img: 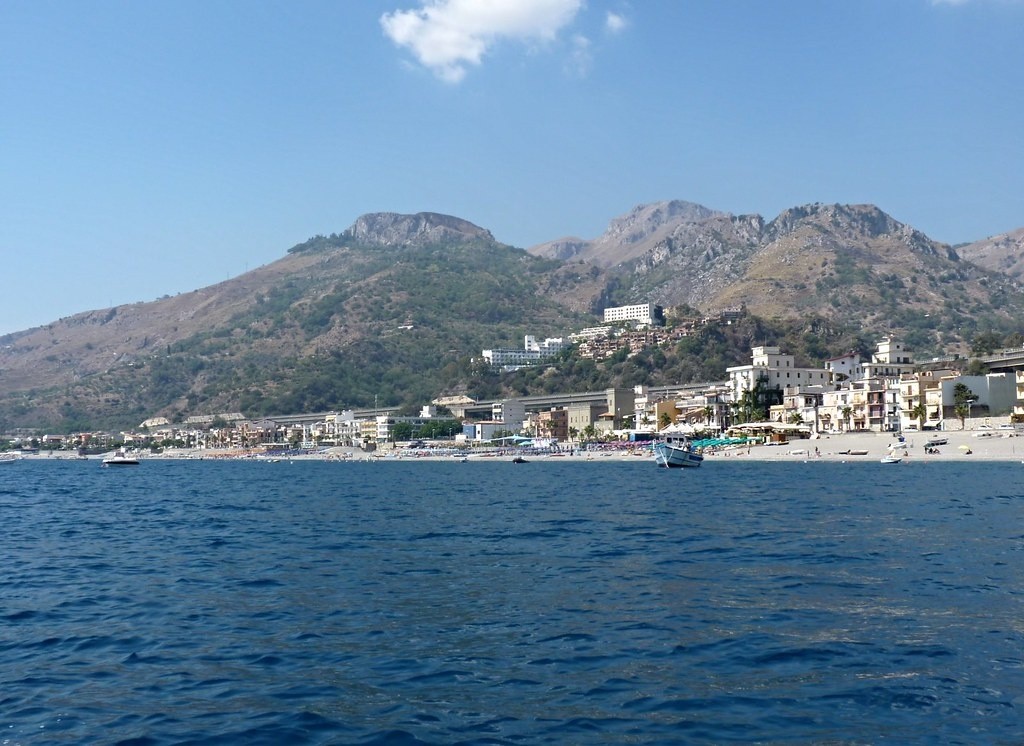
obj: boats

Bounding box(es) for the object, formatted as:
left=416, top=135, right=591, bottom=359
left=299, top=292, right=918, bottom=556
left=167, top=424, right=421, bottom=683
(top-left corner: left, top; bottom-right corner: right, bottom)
left=838, top=449, right=870, bottom=455
left=881, top=456, right=902, bottom=465
left=652, top=439, right=704, bottom=467
left=924, top=436, right=947, bottom=447
left=102, top=450, right=142, bottom=467
left=0, top=451, right=18, bottom=464
left=887, top=441, right=906, bottom=451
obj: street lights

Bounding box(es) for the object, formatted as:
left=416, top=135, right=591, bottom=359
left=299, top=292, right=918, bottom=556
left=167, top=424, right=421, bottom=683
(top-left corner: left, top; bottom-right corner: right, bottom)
left=967, top=399, right=974, bottom=429
left=618, top=407, right=621, bottom=430
left=432, top=428, right=436, bottom=440
left=449, top=428, right=453, bottom=440
left=411, top=430, right=414, bottom=440
